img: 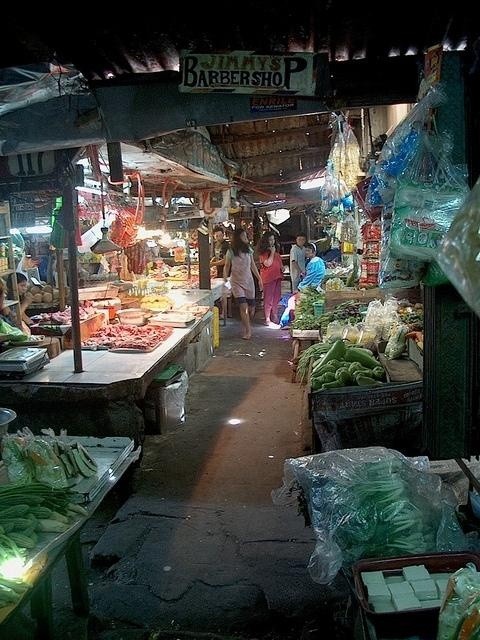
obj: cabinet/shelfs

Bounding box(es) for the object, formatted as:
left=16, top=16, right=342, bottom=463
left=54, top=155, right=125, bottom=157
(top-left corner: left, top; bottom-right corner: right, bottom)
left=0, top=199, right=22, bottom=344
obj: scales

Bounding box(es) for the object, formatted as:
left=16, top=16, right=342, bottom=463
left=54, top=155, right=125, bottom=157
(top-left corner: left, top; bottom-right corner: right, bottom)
left=0, top=346, right=49, bottom=378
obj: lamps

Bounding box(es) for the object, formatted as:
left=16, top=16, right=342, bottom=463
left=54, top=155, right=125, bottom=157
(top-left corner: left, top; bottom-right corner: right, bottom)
left=89, top=174, right=123, bottom=257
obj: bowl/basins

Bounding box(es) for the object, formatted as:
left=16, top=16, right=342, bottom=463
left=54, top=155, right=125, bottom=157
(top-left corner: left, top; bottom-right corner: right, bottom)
left=0, top=406, right=18, bottom=438
left=114, top=308, right=152, bottom=326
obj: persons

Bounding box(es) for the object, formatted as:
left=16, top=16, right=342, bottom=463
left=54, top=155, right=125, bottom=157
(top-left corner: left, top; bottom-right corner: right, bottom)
left=289, top=232, right=308, bottom=294
left=209, top=228, right=233, bottom=319
left=7, top=273, right=34, bottom=326
left=222, top=229, right=264, bottom=340
left=0, top=277, right=27, bottom=343
left=280, top=243, right=326, bottom=330
left=257, top=232, right=285, bottom=325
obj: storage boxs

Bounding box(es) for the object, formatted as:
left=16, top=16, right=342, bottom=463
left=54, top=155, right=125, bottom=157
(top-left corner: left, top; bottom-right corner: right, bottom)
left=30, top=311, right=104, bottom=349
left=351, top=549, right=480, bottom=631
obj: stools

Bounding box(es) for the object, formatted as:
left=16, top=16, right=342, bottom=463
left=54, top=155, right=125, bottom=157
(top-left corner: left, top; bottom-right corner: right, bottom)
left=291, top=328, right=321, bottom=383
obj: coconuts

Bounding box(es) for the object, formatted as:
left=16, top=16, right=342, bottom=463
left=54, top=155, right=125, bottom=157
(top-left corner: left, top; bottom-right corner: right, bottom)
left=30, top=284, right=59, bottom=303
